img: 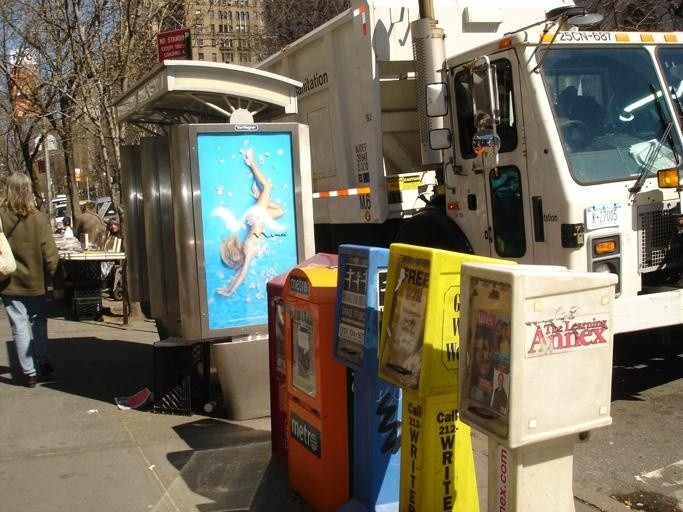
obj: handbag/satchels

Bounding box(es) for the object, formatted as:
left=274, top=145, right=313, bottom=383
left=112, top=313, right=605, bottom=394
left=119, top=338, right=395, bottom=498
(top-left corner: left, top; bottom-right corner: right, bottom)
left=0, top=232, right=17, bottom=282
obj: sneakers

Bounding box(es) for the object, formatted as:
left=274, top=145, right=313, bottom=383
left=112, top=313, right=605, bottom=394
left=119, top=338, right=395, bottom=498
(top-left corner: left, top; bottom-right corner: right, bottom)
left=25, top=373, right=38, bottom=388
left=39, top=364, right=54, bottom=376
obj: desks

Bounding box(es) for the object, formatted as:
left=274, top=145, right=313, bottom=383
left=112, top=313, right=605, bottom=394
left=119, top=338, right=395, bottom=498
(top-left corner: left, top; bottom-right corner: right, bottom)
left=46, top=234, right=129, bottom=326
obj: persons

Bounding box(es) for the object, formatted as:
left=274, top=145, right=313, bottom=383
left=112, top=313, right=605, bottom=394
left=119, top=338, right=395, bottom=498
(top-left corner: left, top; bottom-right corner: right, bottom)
left=106, top=219, right=121, bottom=238
left=73, top=203, right=105, bottom=247
left=63, top=217, right=73, bottom=240
left=0, top=171, right=60, bottom=388
left=216, top=147, right=284, bottom=297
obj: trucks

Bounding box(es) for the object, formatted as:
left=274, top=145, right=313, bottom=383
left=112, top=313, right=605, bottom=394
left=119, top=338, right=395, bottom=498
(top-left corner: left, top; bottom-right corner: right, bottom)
left=253, top=0, right=683, bottom=335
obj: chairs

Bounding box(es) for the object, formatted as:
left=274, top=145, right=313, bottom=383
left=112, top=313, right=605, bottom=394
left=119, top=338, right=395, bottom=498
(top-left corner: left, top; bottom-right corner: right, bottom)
left=74, top=231, right=123, bottom=301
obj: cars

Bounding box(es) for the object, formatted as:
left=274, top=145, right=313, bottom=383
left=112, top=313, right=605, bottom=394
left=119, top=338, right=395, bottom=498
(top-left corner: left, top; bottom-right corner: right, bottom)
left=51, top=195, right=120, bottom=228
